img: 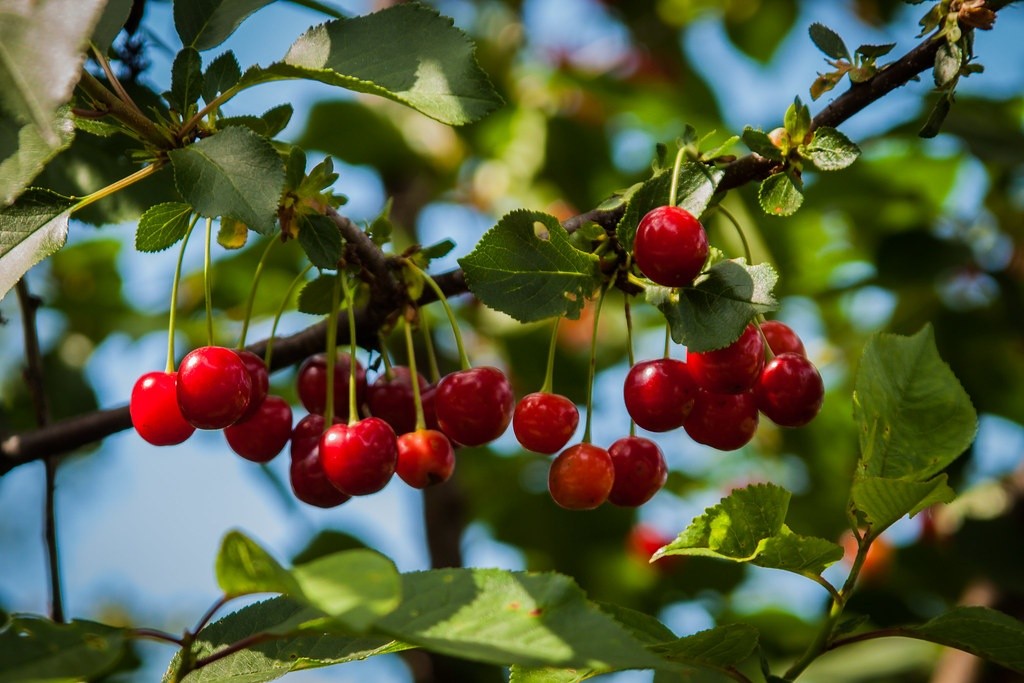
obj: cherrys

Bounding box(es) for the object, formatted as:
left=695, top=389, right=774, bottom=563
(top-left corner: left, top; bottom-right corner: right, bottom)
left=129, top=146, right=825, bottom=511
